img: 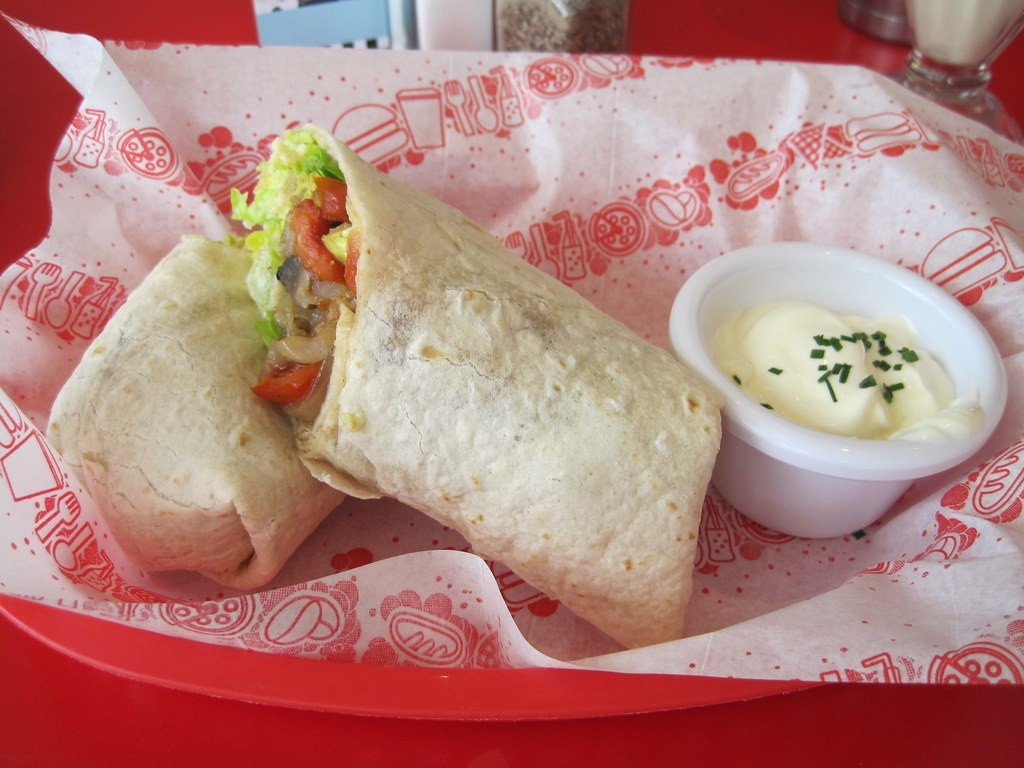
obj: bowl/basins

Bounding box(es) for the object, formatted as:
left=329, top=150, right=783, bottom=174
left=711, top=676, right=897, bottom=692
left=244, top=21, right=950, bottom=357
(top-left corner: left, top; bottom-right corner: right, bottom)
left=670, top=234, right=1007, bottom=538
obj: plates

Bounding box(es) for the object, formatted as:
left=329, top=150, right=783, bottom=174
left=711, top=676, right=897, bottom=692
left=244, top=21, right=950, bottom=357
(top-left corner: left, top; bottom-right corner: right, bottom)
left=0, top=584, right=829, bottom=720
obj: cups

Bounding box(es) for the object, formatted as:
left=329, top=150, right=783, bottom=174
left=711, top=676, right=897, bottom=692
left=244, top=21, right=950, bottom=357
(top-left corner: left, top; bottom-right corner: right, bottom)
left=882, top=1, right=1024, bottom=146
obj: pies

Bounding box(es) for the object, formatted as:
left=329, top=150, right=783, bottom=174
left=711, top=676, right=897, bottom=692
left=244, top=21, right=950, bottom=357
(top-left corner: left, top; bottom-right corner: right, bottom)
left=221, top=122, right=725, bottom=649
left=48, top=232, right=352, bottom=588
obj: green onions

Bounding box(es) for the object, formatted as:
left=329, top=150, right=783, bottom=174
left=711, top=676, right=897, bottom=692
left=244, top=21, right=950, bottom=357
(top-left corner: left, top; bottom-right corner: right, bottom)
left=733, top=331, right=922, bottom=413
